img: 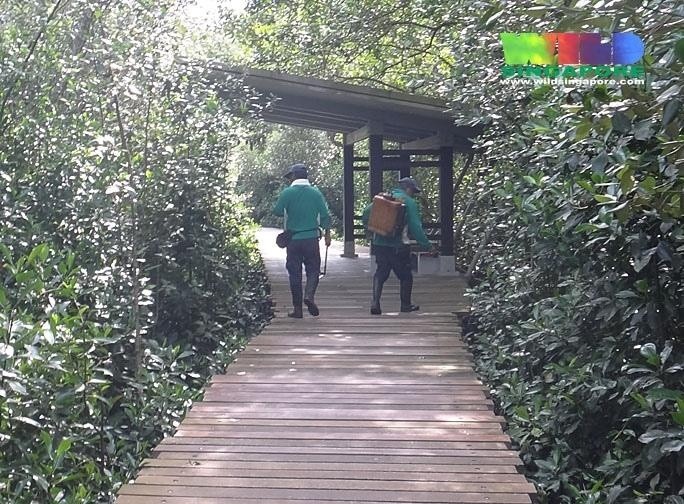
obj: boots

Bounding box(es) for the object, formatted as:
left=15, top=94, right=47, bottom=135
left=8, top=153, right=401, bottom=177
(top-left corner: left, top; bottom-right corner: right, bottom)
left=288, top=304, right=303, bottom=318
left=400, top=280, right=420, bottom=312
left=370, top=280, right=382, bottom=315
left=304, top=286, right=319, bottom=316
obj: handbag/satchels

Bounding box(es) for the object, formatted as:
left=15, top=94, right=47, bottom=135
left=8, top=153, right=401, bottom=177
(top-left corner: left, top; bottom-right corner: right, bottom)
left=276, top=230, right=292, bottom=248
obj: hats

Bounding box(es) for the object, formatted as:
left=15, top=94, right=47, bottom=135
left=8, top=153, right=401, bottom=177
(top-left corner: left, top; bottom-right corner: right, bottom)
left=284, top=164, right=307, bottom=179
left=398, top=177, right=421, bottom=193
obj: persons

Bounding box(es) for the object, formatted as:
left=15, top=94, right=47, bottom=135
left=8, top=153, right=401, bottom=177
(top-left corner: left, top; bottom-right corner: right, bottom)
left=270, top=162, right=331, bottom=318
left=360, top=175, right=441, bottom=315
